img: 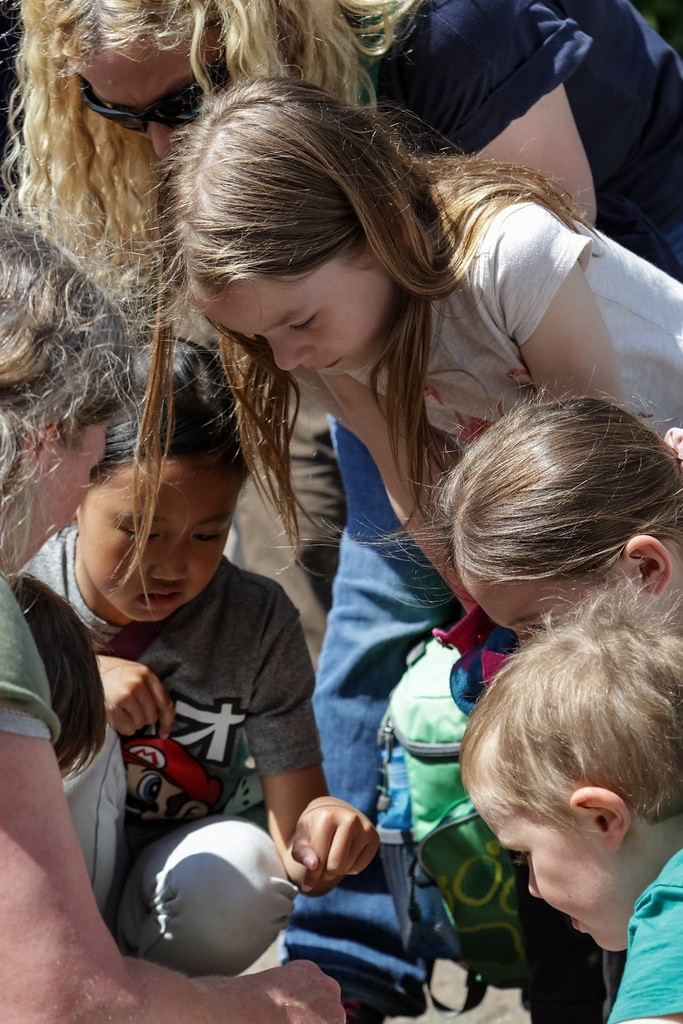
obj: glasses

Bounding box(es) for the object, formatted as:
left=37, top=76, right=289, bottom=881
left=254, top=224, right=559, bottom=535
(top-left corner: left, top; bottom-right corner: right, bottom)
left=73, top=68, right=223, bottom=136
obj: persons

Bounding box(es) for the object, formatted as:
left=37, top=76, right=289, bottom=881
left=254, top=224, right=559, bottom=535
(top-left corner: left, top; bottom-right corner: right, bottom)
left=0, top=0, right=683, bottom=1024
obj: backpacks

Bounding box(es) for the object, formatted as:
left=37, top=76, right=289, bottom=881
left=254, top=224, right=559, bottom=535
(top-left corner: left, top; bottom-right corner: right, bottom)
left=375, top=635, right=543, bottom=991
